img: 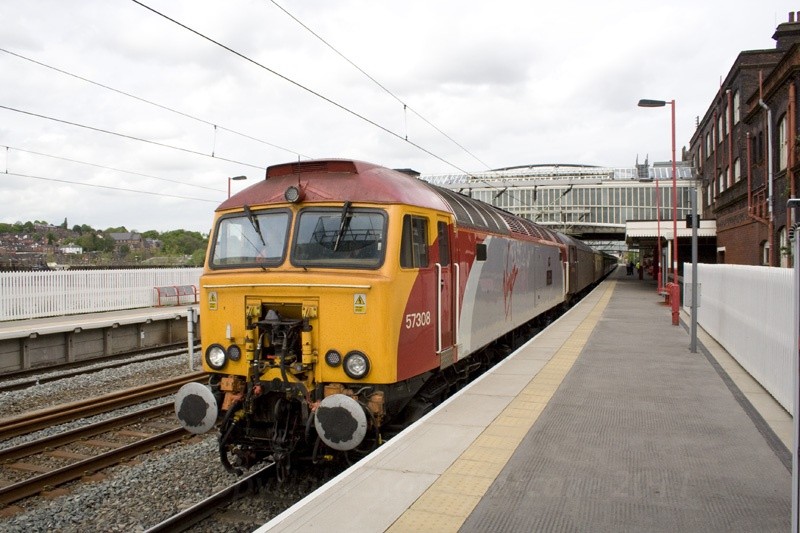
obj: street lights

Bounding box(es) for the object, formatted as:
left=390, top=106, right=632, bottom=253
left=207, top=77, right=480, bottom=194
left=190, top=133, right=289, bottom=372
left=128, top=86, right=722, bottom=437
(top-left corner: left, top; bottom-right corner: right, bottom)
left=228, top=176, right=247, bottom=200
left=638, top=98, right=681, bottom=325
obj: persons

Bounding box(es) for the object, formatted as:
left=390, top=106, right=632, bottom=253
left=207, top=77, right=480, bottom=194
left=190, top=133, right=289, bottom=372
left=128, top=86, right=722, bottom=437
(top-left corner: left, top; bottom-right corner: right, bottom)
left=256, top=228, right=282, bottom=261
left=628, top=260, right=636, bottom=276
left=637, top=265, right=645, bottom=280
left=635, top=259, right=640, bottom=273
left=626, top=262, right=630, bottom=275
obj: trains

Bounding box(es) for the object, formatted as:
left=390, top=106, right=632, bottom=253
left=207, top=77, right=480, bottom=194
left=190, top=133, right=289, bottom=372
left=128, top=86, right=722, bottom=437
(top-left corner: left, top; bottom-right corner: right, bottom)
left=174, top=157, right=620, bottom=484
